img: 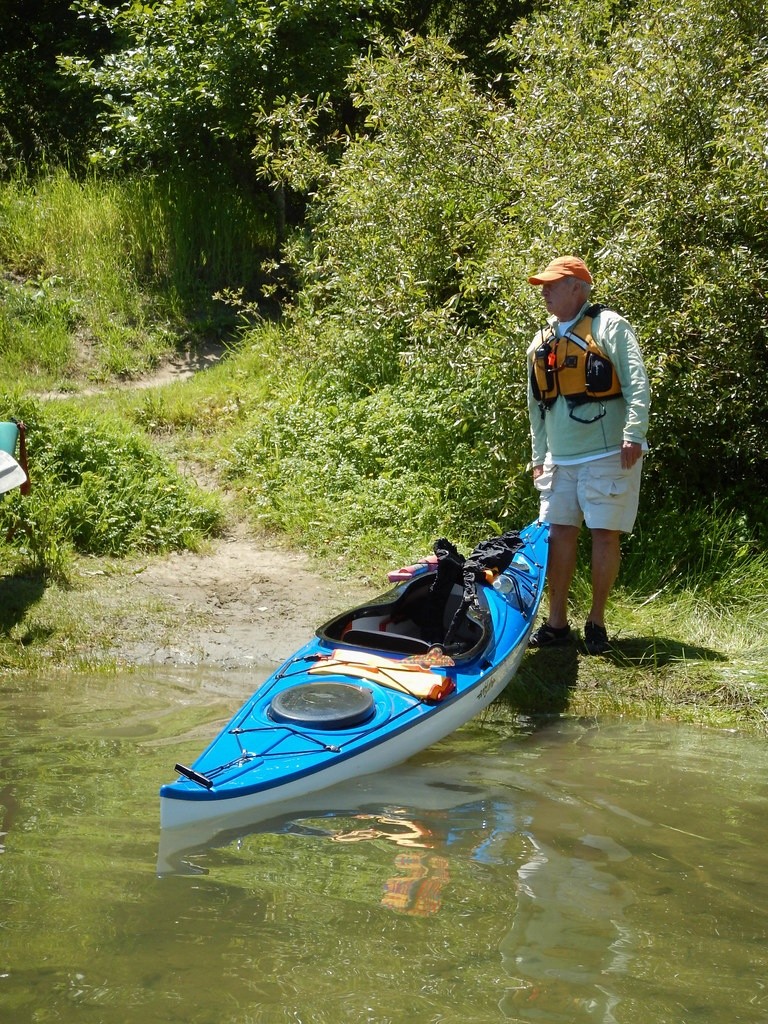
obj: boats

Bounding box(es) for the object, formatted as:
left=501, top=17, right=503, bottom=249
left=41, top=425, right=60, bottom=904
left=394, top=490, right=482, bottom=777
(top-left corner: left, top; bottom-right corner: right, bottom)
left=156, top=516, right=551, bottom=824
left=0, top=421, right=28, bottom=498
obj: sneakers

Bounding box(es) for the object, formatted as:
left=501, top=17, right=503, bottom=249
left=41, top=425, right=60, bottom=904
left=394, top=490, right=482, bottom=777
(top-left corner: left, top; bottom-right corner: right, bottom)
left=584, top=622, right=610, bottom=657
left=527, top=617, right=572, bottom=648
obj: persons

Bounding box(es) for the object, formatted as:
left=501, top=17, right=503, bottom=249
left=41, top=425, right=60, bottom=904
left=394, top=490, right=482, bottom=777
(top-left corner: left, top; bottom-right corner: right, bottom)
left=526, top=256, right=650, bottom=656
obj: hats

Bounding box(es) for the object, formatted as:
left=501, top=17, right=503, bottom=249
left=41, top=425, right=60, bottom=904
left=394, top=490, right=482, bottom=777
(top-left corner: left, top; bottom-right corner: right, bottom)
left=529, top=256, right=592, bottom=285
